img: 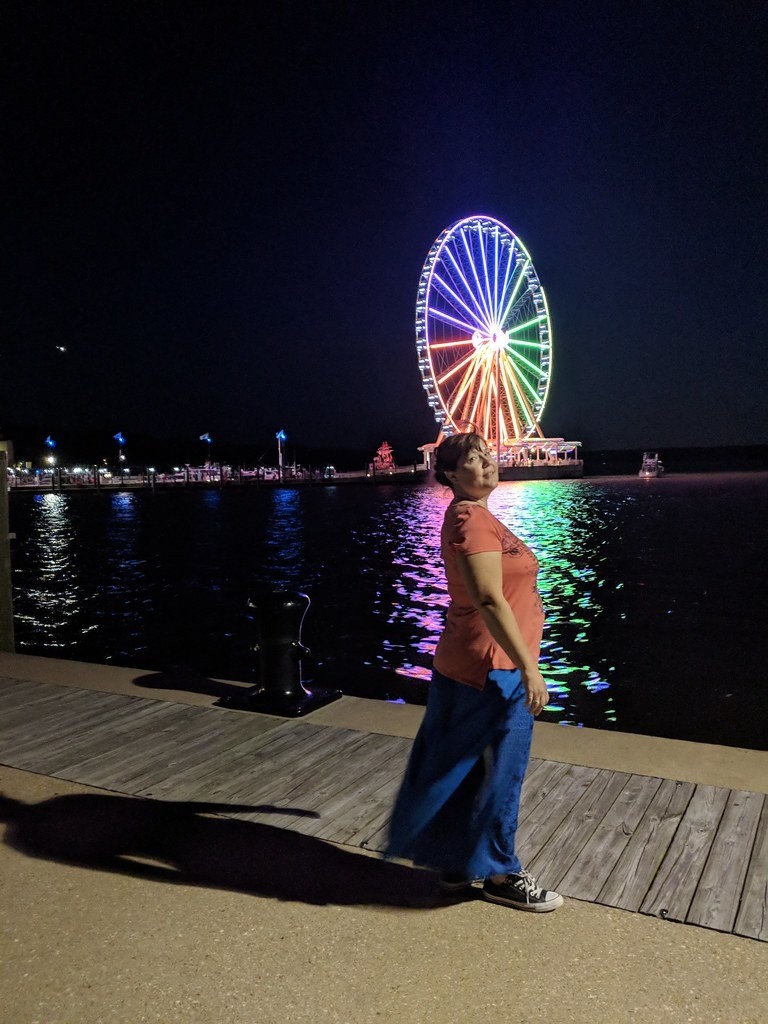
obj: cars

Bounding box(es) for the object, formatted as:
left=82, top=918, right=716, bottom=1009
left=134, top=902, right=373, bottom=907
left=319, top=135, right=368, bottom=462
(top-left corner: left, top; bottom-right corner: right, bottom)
left=321, top=465, right=336, bottom=475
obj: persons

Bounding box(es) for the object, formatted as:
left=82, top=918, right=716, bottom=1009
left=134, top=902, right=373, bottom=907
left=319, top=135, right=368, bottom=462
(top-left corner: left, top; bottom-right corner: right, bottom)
left=387, top=431, right=564, bottom=912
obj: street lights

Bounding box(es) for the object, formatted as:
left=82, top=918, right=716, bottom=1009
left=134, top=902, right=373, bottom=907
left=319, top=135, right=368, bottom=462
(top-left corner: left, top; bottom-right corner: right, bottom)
left=113, top=432, right=125, bottom=486
left=276, top=429, right=286, bottom=483
left=199, top=432, right=211, bottom=481
left=46, top=435, right=55, bottom=490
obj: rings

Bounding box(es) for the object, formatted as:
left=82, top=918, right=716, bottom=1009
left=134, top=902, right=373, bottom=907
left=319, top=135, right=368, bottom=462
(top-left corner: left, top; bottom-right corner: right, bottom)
left=533, top=700, right=540, bottom=704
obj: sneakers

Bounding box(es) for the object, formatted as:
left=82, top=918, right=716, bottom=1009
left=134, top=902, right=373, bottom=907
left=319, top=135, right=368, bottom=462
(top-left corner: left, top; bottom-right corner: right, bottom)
left=441, top=878, right=484, bottom=889
left=482, top=867, right=563, bottom=912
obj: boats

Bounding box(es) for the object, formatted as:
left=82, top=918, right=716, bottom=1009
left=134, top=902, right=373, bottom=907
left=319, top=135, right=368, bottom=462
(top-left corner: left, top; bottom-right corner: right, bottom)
left=639, top=452, right=664, bottom=478
